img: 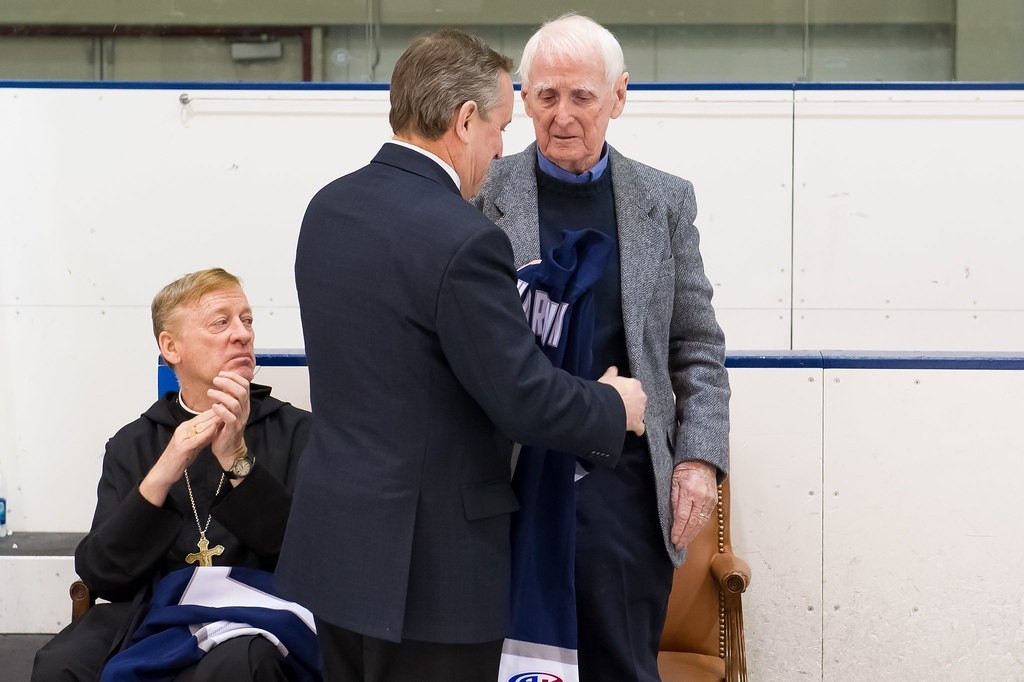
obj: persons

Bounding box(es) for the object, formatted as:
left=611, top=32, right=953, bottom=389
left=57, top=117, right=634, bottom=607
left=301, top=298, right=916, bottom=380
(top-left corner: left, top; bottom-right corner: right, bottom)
left=32, top=269, right=315, bottom=682
left=462, top=11, right=732, bottom=682
left=293, top=31, right=648, bottom=682
left=183, top=467, right=225, bottom=568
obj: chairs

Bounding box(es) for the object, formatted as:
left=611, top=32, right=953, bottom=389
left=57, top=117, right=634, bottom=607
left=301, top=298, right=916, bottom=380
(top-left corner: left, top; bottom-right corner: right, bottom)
left=658, top=477, right=752, bottom=682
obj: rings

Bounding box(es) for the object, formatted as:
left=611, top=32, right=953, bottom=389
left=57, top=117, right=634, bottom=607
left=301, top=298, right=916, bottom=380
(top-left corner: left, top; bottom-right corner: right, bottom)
left=700, top=512, right=709, bottom=519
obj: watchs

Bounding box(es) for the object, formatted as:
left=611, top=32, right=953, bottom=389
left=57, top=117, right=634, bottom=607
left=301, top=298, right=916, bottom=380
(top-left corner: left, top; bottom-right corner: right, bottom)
left=224, top=450, right=257, bottom=479
